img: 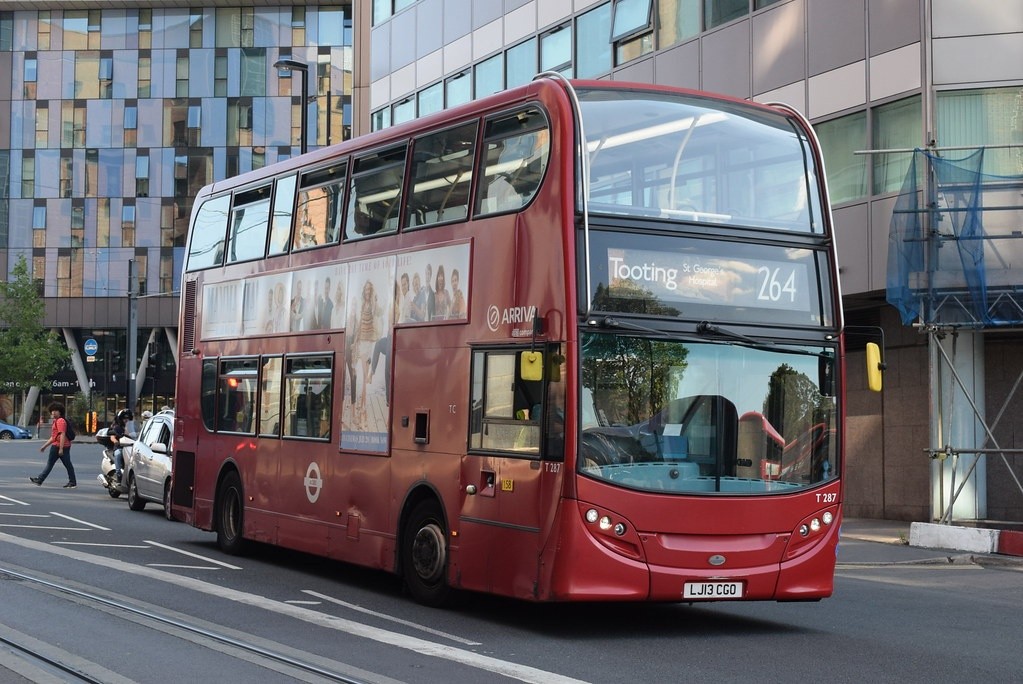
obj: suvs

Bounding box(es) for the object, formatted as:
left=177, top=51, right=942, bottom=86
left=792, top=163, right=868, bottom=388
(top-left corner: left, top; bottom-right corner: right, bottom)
left=126, top=409, right=178, bottom=521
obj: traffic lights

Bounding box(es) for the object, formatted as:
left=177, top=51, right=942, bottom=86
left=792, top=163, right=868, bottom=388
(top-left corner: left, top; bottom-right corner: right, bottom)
left=108, top=349, right=120, bottom=382
left=227, top=377, right=239, bottom=392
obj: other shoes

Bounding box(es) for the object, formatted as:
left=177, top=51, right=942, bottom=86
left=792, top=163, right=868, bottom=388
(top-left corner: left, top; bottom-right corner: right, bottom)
left=117, top=477, right=122, bottom=486
left=63, top=483, right=77, bottom=488
left=30, top=477, right=42, bottom=486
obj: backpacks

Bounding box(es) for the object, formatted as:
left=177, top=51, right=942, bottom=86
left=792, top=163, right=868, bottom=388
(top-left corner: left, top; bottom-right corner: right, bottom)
left=60, top=417, right=76, bottom=442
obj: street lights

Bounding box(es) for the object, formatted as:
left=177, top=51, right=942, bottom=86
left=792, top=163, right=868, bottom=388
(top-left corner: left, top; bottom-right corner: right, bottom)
left=273, top=59, right=309, bottom=154
left=126, top=258, right=140, bottom=410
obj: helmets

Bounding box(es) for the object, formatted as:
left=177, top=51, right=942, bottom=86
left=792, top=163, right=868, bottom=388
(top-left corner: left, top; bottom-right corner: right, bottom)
left=118, top=409, right=133, bottom=426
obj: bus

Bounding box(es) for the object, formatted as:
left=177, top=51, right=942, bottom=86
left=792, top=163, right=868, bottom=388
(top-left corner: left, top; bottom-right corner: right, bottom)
left=168, top=69, right=887, bottom=608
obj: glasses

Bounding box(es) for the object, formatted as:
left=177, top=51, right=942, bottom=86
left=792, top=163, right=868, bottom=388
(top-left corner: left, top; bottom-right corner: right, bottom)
left=122, top=417, right=129, bottom=420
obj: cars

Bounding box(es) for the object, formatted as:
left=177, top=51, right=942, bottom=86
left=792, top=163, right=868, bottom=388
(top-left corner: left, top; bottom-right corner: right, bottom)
left=0, top=420, right=33, bottom=439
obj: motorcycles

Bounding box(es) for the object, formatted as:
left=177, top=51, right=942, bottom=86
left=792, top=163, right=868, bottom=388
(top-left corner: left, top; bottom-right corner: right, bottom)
left=96, top=419, right=142, bottom=499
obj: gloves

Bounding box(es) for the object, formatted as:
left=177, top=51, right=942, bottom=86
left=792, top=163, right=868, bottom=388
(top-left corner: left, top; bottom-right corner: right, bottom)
left=115, top=441, right=121, bottom=449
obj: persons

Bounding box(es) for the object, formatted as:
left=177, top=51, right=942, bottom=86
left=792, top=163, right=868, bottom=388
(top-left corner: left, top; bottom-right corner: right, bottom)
left=261, top=264, right=465, bottom=417
left=533, top=381, right=563, bottom=442
left=315, top=383, right=330, bottom=438
left=29, top=402, right=78, bottom=489
left=107, top=408, right=153, bottom=486
